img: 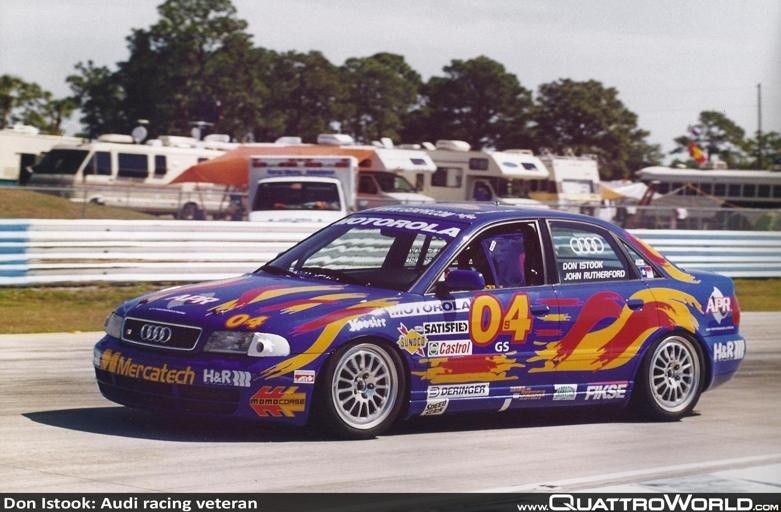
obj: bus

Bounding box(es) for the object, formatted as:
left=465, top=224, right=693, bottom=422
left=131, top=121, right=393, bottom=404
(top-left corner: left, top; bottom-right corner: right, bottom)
left=633, top=164, right=781, bottom=215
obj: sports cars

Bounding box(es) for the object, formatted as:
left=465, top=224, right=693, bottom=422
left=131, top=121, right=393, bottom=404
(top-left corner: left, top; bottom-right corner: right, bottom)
left=88, top=200, right=746, bottom=439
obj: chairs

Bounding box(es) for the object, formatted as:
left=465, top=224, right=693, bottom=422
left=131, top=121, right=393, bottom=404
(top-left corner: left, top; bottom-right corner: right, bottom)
left=448, top=268, right=484, bottom=294
